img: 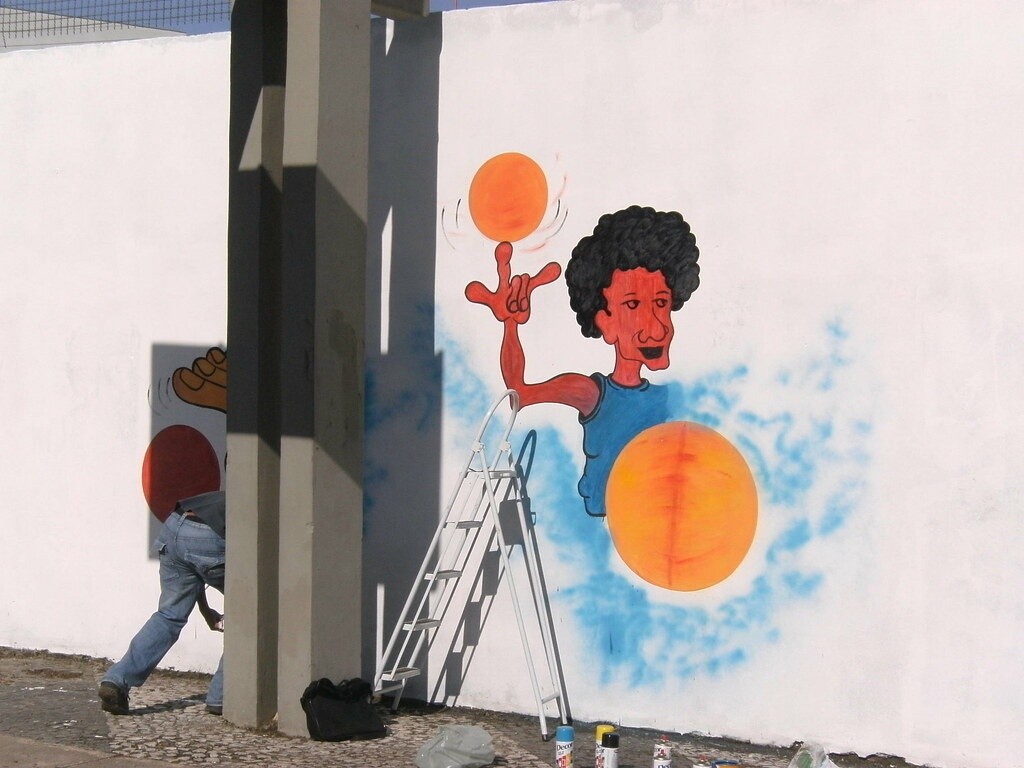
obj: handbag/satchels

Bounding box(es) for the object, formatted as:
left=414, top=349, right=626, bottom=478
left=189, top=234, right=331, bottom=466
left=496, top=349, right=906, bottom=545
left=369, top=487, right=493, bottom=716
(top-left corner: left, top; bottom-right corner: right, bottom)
left=298, top=674, right=386, bottom=741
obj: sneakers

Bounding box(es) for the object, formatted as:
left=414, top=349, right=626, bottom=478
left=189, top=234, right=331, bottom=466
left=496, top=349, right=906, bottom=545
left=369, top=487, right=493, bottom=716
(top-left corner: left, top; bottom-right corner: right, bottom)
left=98, top=683, right=129, bottom=715
left=205, top=704, right=225, bottom=715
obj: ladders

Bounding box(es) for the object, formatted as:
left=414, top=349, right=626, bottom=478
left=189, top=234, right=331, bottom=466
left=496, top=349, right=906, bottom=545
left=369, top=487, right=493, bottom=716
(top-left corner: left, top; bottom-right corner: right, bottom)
left=368, top=389, right=573, bottom=744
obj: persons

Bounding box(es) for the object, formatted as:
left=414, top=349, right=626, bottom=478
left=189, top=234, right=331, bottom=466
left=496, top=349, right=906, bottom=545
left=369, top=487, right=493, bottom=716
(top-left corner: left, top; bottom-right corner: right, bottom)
left=98, top=490, right=228, bottom=714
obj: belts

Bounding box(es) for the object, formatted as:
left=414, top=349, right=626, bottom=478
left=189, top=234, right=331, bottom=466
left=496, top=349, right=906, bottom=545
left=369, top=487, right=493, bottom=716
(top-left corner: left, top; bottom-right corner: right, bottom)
left=174, top=509, right=206, bottom=523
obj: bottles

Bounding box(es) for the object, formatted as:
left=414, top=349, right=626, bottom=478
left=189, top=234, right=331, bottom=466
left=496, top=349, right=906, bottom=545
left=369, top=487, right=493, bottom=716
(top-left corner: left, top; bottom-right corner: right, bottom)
left=594, top=724, right=614, bottom=768
left=692, top=755, right=741, bottom=767
left=654, top=754, right=671, bottom=768
left=654, top=735, right=672, bottom=759
left=555, top=726, right=574, bottom=768
left=601, top=732, right=619, bottom=768
